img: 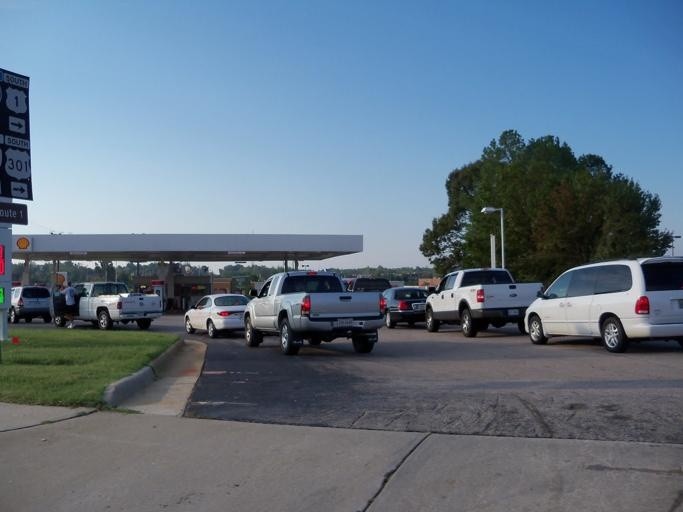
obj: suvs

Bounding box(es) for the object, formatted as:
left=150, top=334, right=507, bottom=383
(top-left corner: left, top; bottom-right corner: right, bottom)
left=7, top=286, right=51, bottom=324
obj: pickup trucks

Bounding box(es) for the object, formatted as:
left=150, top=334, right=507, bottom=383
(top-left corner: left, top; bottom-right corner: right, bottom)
left=423, top=268, right=544, bottom=337
left=49, top=281, right=163, bottom=330
left=241, top=270, right=385, bottom=356
left=340, top=277, right=392, bottom=293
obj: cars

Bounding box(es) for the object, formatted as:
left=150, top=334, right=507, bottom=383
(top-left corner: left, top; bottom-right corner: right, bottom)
left=381, top=287, right=430, bottom=329
left=183, top=294, right=250, bottom=339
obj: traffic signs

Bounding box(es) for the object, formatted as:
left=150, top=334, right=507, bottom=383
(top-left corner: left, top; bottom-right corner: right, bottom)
left=0, top=202, right=29, bottom=226
left=0, top=65, right=32, bottom=200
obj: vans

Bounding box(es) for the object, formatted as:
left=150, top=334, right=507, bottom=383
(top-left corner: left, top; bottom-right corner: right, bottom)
left=523, top=256, right=682, bottom=353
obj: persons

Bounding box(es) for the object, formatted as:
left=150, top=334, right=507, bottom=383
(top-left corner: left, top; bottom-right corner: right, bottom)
left=59, top=280, right=77, bottom=329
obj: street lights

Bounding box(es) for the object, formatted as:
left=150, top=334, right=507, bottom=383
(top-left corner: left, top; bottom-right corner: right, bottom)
left=670, top=235, right=680, bottom=254
left=481, top=207, right=504, bottom=270
